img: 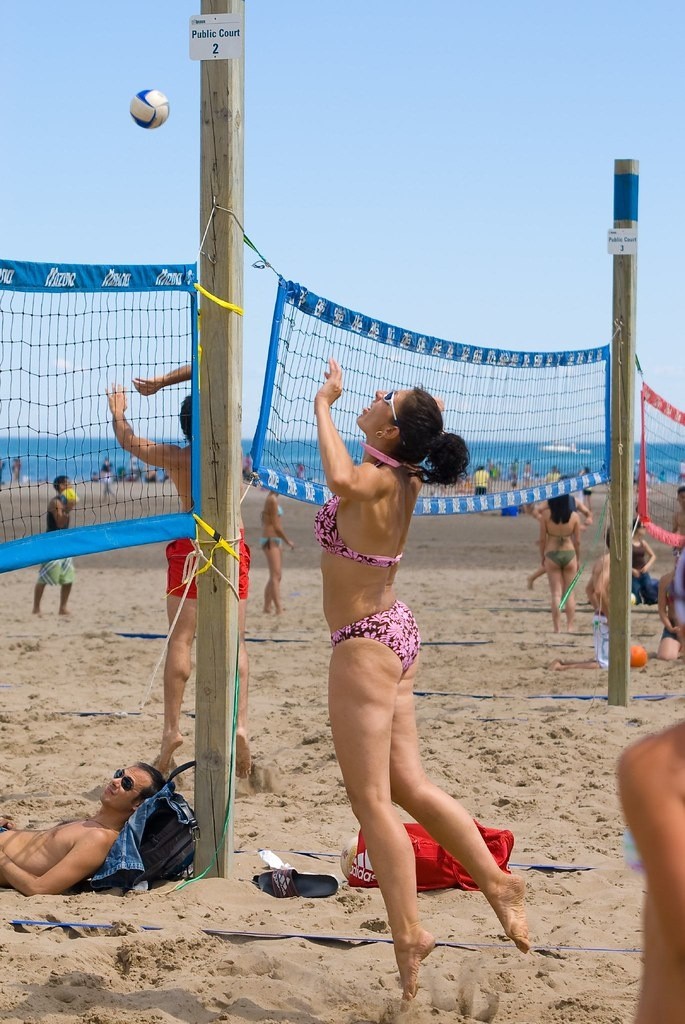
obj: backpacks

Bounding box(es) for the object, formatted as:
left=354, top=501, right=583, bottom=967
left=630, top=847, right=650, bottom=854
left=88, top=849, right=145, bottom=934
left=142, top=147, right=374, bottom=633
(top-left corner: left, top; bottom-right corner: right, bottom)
left=70, top=807, right=196, bottom=894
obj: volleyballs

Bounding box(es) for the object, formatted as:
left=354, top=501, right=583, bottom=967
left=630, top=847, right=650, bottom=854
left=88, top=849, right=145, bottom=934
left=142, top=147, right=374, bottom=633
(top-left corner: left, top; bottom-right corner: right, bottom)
left=130, top=89, right=172, bottom=131
left=61, top=487, right=79, bottom=506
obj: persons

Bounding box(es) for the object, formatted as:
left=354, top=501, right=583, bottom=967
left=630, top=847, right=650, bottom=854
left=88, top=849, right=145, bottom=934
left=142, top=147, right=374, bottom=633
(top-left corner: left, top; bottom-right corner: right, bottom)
left=33, top=476, right=80, bottom=614
left=671, top=487, right=685, bottom=565
left=257, top=489, right=296, bottom=616
left=540, top=495, right=582, bottom=633
left=617, top=719, right=685, bottom=1024
left=0, top=760, right=167, bottom=898
left=313, top=359, right=530, bottom=1001
left=587, top=517, right=685, bottom=659
left=106, top=362, right=252, bottom=778
left=0, top=452, right=685, bottom=517
left=526, top=475, right=593, bottom=591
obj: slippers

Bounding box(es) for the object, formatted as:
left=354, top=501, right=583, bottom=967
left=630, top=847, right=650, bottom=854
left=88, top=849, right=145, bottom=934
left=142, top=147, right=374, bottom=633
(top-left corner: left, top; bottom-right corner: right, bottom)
left=258, top=870, right=339, bottom=898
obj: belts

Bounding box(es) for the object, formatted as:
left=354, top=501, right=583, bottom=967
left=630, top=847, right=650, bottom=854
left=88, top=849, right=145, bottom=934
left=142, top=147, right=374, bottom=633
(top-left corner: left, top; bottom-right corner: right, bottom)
left=166, top=760, right=201, bottom=843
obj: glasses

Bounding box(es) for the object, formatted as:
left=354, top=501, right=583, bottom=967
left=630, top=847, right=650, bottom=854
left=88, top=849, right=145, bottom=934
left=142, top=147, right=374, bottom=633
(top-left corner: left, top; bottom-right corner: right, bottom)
left=383, top=390, right=405, bottom=446
left=114, top=769, right=149, bottom=798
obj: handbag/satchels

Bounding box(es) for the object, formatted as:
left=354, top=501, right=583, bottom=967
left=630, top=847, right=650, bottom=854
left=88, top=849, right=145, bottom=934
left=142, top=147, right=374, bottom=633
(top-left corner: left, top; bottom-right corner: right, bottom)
left=348, top=818, right=515, bottom=891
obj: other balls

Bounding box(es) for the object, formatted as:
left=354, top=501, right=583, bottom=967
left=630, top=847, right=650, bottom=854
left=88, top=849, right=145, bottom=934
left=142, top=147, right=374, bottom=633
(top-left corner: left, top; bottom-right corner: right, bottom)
left=630, top=642, right=648, bottom=666
